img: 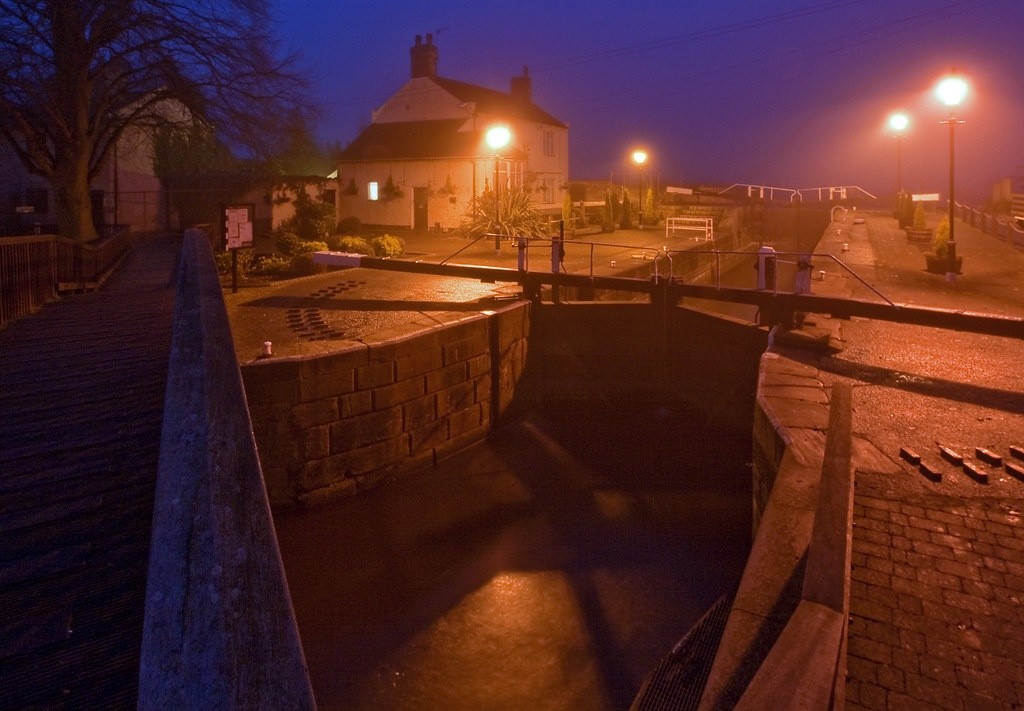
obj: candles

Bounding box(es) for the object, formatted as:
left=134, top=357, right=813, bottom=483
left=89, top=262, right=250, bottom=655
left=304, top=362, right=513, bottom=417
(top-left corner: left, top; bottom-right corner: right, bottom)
left=893, top=192, right=914, bottom=229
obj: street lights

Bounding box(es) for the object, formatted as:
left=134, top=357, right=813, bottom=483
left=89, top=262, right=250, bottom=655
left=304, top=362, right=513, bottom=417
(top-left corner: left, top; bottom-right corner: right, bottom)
left=632, top=151, right=647, bottom=225
left=931, top=76, right=970, bottom=271
left=889, top=113, right=908, bottom=219
left=486, top=126, right=511, bottom=250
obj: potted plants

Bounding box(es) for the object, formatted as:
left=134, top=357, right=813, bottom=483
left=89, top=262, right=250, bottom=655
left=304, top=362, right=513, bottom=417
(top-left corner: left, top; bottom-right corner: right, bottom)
left=643, top=188, right=660, bottom=226
left=904, top=199, right=933, bottom=242
left=620, top=191, right=632, bottom=230
left=925, top=214, right=963, bottom=274
left=556, top=189, right=574, bottom=239
left=602, top=192, right=615, bottom=233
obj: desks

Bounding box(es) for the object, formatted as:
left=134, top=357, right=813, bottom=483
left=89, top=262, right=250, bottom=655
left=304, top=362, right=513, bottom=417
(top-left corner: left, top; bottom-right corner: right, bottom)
left=666, top=218, right=713, bottom=241
